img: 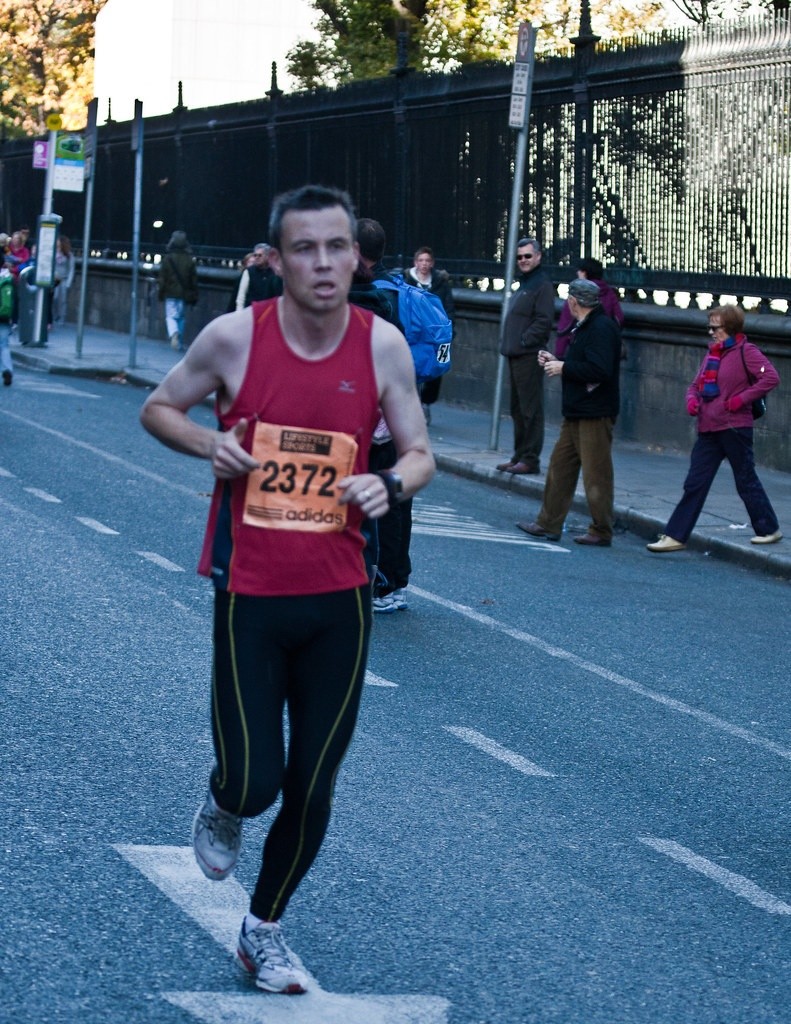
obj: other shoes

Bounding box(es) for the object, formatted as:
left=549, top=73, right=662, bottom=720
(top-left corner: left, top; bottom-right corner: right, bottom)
left=647, top=533, right=685, bottom=551
left=751, top=530, right=782, bottom=544
left=3, top=370, right=11, bottom=385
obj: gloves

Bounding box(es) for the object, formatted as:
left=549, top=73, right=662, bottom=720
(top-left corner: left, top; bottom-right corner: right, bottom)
left=687, top=397, right=699, bottom=415
left=724, top=396, right=743, bottom=413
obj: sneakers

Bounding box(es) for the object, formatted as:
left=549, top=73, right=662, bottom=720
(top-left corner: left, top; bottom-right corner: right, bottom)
left=373, top=591, right=407, bottom=612
left=192, top=791, right=242, bottom=880
left=235, top=916, right=307, bottom=994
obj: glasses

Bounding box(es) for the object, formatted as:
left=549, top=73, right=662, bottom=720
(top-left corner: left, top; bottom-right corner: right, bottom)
left=706, top=325, right=723, bottom=331
left=517, top=253, right=537, bottom=260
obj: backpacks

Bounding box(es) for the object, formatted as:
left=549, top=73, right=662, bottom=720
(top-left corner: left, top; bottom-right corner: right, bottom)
left=369, top=274, right=453, bottom=384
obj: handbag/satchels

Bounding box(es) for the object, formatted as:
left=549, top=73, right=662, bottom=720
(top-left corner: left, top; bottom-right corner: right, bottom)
left=184, top=288, right=196, bottom=303
left=741, top=344, right=765, bottom=420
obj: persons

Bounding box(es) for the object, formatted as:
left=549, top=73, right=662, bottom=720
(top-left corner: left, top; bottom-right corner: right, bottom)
left=0, top=227, right=58, bottom=386
left=400, top=248, right=455, bottom=423
left=516, top=278, right=622, bottom=546
left=349, top=218, right=407, bottom=614
left=139, top=183, right=436, bottom=992
left=229, top=243, right=284, bottom=314
left=557, top=258, right=624, bottom=361
left=647, top=304, right=782, bottom=552
left=158, top=230, right=199, bottom=348
left=53, top=236, right=74, bottom=329
left=496, top=238, right=555, bottom=473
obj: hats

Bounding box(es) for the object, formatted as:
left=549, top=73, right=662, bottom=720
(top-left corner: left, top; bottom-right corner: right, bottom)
left=569, top=279, right=600, bottom=306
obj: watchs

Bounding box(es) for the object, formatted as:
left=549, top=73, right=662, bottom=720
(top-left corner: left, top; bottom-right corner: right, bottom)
left=375, top=470, right=403, bottom=507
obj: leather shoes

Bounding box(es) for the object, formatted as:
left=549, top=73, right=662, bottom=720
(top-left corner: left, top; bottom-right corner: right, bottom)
left=506, top=462, right=539, bottom=473
left=496, top=462, right=515, bottom=470
left=516, top=522, right=560, bottom=540
left=574, top=533, right=610, bottom=545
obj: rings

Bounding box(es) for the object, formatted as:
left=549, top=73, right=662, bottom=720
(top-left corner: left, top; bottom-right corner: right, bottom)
left=364, top=491, right=370, bottom=499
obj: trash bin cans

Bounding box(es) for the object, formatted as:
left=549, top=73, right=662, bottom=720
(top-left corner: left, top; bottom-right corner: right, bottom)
left=15, top=265, right=53, bottom=349
